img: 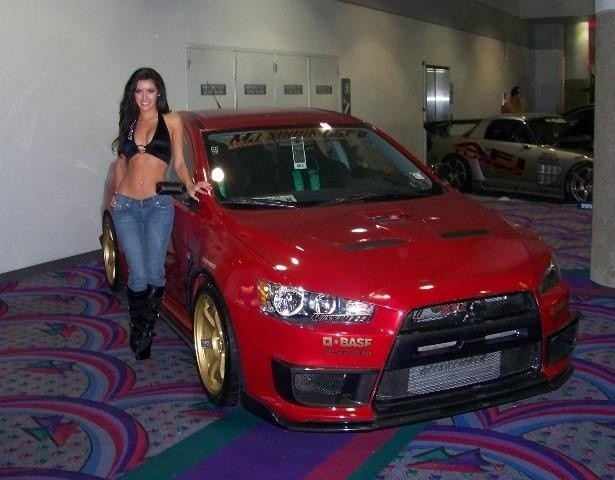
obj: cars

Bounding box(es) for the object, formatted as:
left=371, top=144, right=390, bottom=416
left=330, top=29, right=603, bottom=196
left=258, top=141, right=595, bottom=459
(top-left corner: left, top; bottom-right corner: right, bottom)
left=97, top=105, right=583, bottom=434
left=433, top=104, right=592, bottom=208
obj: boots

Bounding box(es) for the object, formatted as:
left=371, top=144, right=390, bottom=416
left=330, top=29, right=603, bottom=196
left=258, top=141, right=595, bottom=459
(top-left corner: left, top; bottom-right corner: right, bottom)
left=126, top=283, right=165, bottom=360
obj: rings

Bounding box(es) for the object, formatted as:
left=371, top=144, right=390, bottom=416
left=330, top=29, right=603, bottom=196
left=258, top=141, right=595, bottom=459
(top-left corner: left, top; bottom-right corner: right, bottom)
left=195, top=188, right=199, bottom=191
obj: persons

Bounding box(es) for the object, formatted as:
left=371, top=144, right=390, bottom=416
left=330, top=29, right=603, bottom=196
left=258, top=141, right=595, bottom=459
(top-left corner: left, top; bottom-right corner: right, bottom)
left=502, top=86, right=526, bottom=113
left=110, top=68, right=213, bottom=359
